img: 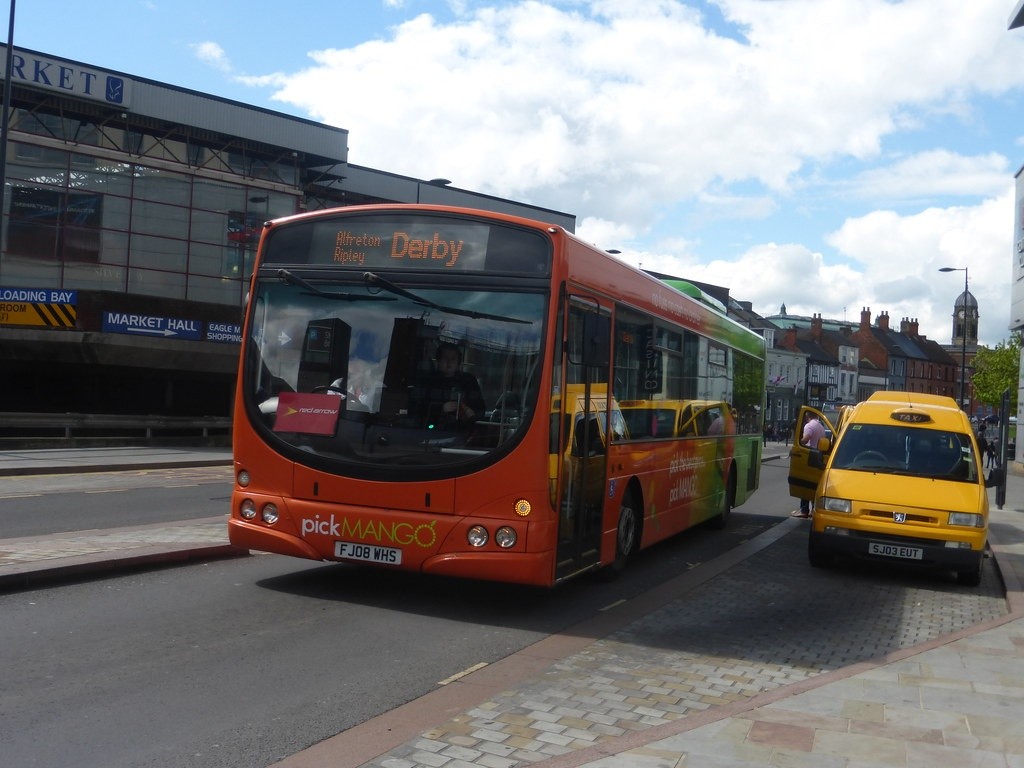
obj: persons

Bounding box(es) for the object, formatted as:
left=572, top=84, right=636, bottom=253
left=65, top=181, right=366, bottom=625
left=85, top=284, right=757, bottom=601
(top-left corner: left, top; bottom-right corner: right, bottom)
left=327, top=340, right=485, bottom=445
left=709, top=403, right=737, bottom=436
left=976, top=424, right=1000, bottom=469
left=790, top=410, right=826, bottom=517
left=611, top=412, right=623, bottom=441
left=767, top=425, right=777, bottom=442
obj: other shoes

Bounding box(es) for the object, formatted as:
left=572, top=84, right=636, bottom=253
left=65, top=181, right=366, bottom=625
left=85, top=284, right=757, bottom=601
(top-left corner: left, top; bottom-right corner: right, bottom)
left=791, top=509, right=809, bottom=519
left=808, top=511, right=812, bottom=517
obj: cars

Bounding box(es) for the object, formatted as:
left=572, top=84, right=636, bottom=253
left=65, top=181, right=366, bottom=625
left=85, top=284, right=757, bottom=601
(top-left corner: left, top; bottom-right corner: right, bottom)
left=788, top=391, right=1004, bottom=585
left=968, top=415, right=978, bottom=423
left=980, top=414, right=999, bottom=424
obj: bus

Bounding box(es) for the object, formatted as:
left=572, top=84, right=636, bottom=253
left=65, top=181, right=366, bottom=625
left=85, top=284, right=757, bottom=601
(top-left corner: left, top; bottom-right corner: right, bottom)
left=228, top=204, right=767, bottom=585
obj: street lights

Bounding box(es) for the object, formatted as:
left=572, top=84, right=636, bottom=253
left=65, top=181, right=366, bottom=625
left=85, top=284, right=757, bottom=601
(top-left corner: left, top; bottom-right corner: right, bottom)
left=884, top=345, right=901, bottom=391
left=939, top=268, right=967, bottom=411
left=417, top=179, right=451, bottom=204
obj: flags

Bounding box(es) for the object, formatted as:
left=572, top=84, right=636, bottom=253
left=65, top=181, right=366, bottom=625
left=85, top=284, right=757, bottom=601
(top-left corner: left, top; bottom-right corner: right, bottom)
left=769, top=376, right=800, bottom=395
